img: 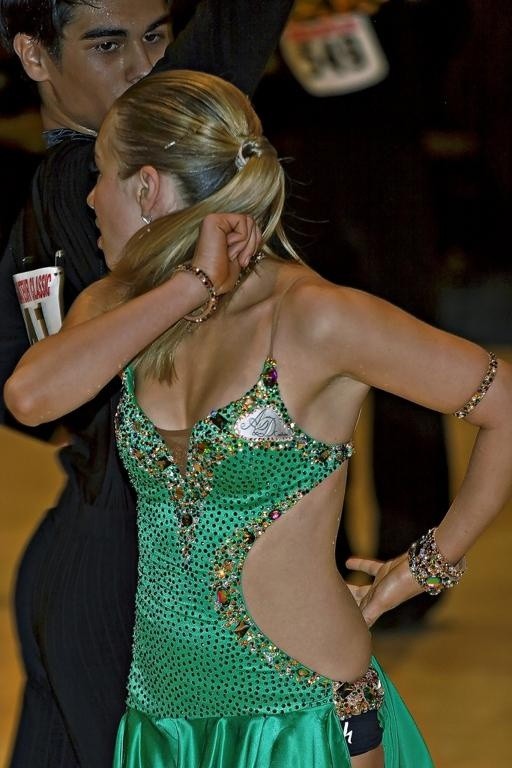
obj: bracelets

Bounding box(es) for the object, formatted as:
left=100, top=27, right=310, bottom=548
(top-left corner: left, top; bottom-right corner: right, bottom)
left=408, top=528, right=463, bottom=596
left=170, top=262, right=218, bottom=321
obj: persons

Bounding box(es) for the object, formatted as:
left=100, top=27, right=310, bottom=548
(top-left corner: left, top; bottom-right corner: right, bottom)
left=2, top=69, right=512, bottom=768
left=1, top=1, right=296, bottom=768
left=250, top=1, right=452, bottom=626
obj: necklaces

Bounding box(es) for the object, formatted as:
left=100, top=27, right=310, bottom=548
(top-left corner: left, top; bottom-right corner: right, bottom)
left=234, top=252, right=265, bottom=286
